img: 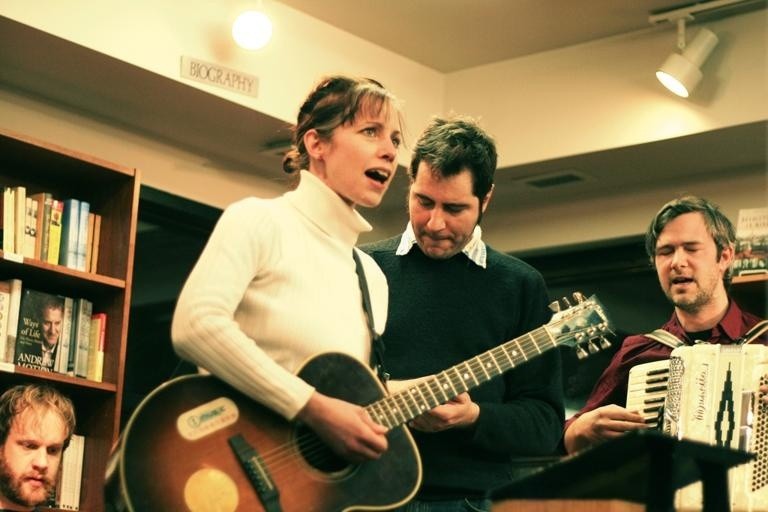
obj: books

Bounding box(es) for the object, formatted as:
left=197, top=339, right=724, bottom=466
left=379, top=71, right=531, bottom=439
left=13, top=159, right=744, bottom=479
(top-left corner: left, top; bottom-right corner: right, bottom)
left=54, top=433, right=87, bottom=511
left=1, top=185, right=108, bottom=383
left=727, top=205, right=767, bottom=276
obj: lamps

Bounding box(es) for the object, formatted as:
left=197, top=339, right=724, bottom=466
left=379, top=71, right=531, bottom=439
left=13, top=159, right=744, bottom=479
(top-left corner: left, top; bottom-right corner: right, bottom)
left=656, top=0, right=720, bottom=97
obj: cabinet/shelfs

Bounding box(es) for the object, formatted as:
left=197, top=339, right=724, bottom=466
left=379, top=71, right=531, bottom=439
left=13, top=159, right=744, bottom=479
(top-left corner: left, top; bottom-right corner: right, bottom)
left=0, top=128, right=142, bottom=512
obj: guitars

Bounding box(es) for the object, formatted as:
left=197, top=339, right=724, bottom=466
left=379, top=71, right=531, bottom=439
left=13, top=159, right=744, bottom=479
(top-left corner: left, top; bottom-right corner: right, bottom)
left=104, top=293, right=617, bottom=512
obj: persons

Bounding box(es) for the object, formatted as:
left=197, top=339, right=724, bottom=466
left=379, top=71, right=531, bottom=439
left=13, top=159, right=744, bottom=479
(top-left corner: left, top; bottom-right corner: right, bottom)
left=356, top=110, right=569, bottom=512
left=561, top=192, right=767, bottom=460
left=0, top=383, right=76, bottom=512
left=168, top=73, right=472, bottom=468
